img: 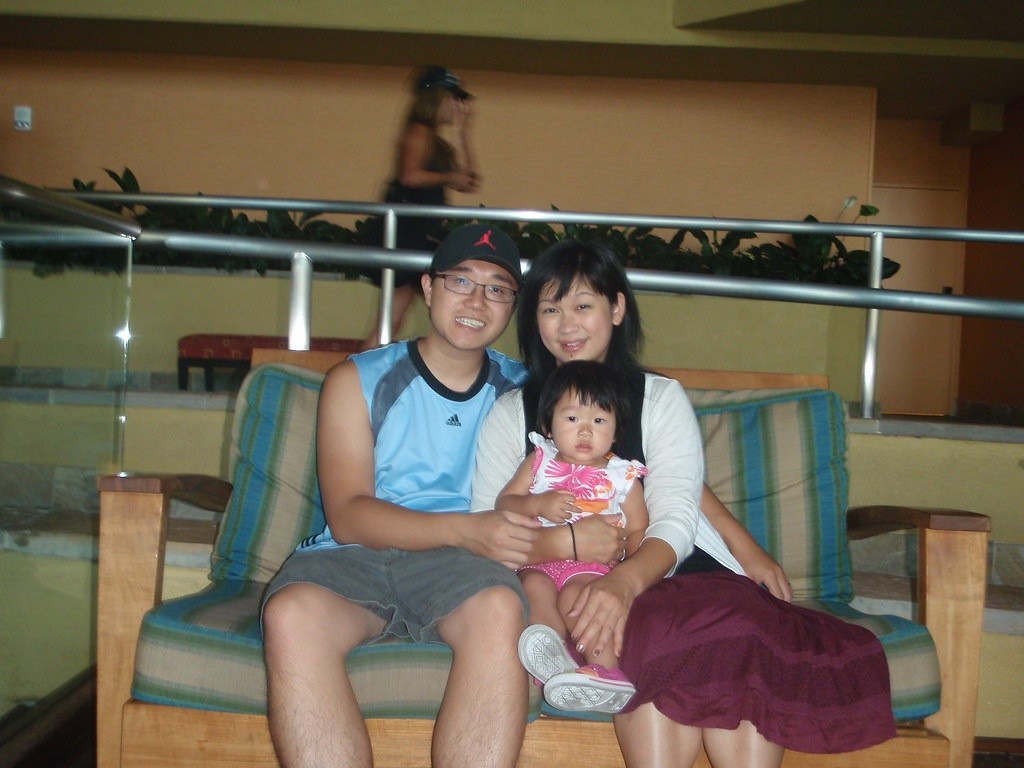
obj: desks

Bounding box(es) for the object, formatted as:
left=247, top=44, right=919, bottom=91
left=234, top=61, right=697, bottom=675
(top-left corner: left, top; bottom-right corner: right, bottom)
left=175, top=332, right=366, bottom=392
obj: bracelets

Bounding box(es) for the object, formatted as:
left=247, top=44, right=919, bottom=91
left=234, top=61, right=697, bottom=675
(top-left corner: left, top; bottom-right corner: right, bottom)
left=568, top=522, right=577, bottom=561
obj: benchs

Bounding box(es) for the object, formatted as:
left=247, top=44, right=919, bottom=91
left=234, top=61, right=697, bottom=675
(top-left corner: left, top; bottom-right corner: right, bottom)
left=94, top=347, right=994, bottom=768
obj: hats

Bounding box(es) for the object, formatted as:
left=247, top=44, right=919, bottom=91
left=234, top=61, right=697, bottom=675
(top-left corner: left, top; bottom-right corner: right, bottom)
left=434, top=223, right=521, bottom=285
left=414, top=63, right=470, bottom=100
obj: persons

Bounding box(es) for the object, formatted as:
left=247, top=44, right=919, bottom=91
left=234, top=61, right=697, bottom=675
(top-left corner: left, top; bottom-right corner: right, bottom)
left=362, top=64, right=480, bottom=350
left=259, top=222, right=898, bottom=768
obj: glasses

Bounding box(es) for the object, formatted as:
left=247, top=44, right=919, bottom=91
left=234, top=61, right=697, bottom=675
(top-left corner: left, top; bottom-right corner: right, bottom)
left=433, top=273, right=517, bottom=303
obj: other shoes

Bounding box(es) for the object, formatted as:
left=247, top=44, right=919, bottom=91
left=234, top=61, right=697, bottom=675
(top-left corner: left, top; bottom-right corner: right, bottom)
left=544, top=662, right=636, bottom=713
left=516, top=624, right=583, bottom=684
